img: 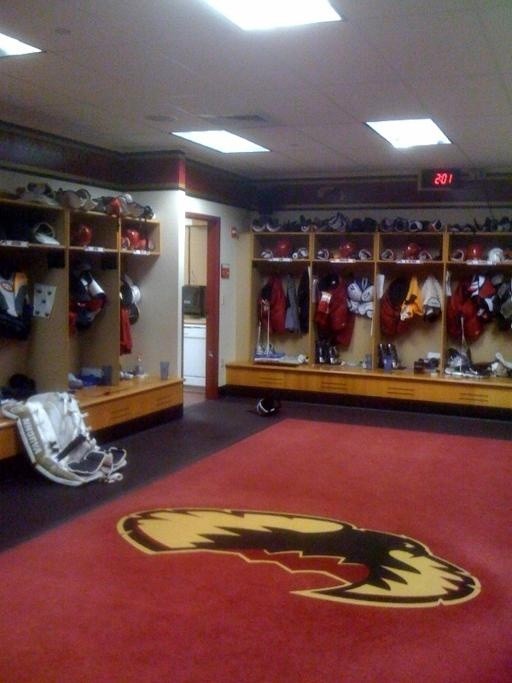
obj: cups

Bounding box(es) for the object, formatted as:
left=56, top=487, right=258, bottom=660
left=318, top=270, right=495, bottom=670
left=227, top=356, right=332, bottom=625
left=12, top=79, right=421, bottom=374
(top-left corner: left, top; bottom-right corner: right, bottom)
left=160, top=360, right=169, bottom=381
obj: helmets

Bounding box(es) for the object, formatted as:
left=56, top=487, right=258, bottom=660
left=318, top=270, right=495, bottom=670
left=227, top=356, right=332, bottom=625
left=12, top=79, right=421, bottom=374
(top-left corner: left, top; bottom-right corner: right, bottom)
left=338, top=237, right=358, bottom=258
left=402, top=240, right=420, bottom=259
left=271, top=237, right=294, bottom=257
left=464, top=241, right=484, bottom=258
left=69, top=221, right=94, bottom=246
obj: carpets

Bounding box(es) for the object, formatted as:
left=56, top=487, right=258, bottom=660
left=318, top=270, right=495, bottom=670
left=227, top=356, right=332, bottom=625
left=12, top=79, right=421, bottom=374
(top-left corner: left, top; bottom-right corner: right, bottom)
left=0, top=416, right=512, bottom=683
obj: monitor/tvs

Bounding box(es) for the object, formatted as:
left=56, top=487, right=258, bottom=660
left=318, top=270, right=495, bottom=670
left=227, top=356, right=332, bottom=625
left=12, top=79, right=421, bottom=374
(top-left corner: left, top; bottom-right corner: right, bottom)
left=182, top=284, right=205, bottom=316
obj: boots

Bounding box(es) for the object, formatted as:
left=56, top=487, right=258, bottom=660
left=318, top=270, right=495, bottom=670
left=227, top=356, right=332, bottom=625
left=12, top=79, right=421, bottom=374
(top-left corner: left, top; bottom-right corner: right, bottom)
left=379, top=343, right=388, bottom=369
left=386, top=343, right=407, bottom=370
left=317, top=341, right=329, bottom=364
left=327, top=344, right=341, bottom=365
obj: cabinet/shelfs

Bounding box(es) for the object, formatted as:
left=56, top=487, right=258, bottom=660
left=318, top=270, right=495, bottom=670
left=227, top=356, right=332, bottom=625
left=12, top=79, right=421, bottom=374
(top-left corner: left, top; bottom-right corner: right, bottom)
left=225, top=227, right=512, bottom=409
left=0, top=198, right=186, bottom=459
